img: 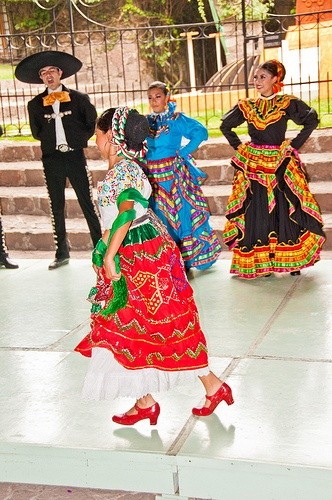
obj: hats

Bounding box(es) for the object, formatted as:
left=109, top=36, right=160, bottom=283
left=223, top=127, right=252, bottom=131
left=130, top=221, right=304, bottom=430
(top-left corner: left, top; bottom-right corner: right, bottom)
left=15, top=51, right=83, bottom=84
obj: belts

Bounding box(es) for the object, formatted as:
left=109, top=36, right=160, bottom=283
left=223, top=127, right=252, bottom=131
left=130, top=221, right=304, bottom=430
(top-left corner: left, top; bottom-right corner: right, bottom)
left=56, top=144, right=75, bottom=152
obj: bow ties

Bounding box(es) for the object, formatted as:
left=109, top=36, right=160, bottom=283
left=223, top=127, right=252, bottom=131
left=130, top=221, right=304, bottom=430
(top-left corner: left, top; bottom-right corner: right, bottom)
left=42, top=91, right=71, bottom=106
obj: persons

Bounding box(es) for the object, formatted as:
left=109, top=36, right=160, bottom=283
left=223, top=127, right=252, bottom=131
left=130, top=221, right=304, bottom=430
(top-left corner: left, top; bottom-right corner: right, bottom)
left=74, top=106, right=234, bottom=426
left=220, top=59, right=326, bottom=280
left=0, top=126, right=18, bottom=269
left=15, top=51, right=102, bottom=269
left=145, top=81, right=222, bottom=274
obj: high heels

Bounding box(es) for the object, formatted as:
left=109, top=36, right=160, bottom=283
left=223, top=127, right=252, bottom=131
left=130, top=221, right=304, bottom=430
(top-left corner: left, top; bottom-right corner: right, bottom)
left=112, top=403, right=160, bottom=425
left=192, top=382, right=234, bottom=417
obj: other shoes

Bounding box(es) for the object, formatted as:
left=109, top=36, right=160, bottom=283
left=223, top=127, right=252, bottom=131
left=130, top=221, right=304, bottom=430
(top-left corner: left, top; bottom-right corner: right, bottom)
left=48, top=258, right=69, bottom=268
left=290, top=270, right=301, bottom=277
left=0, top=258, right=19, bottom=269
